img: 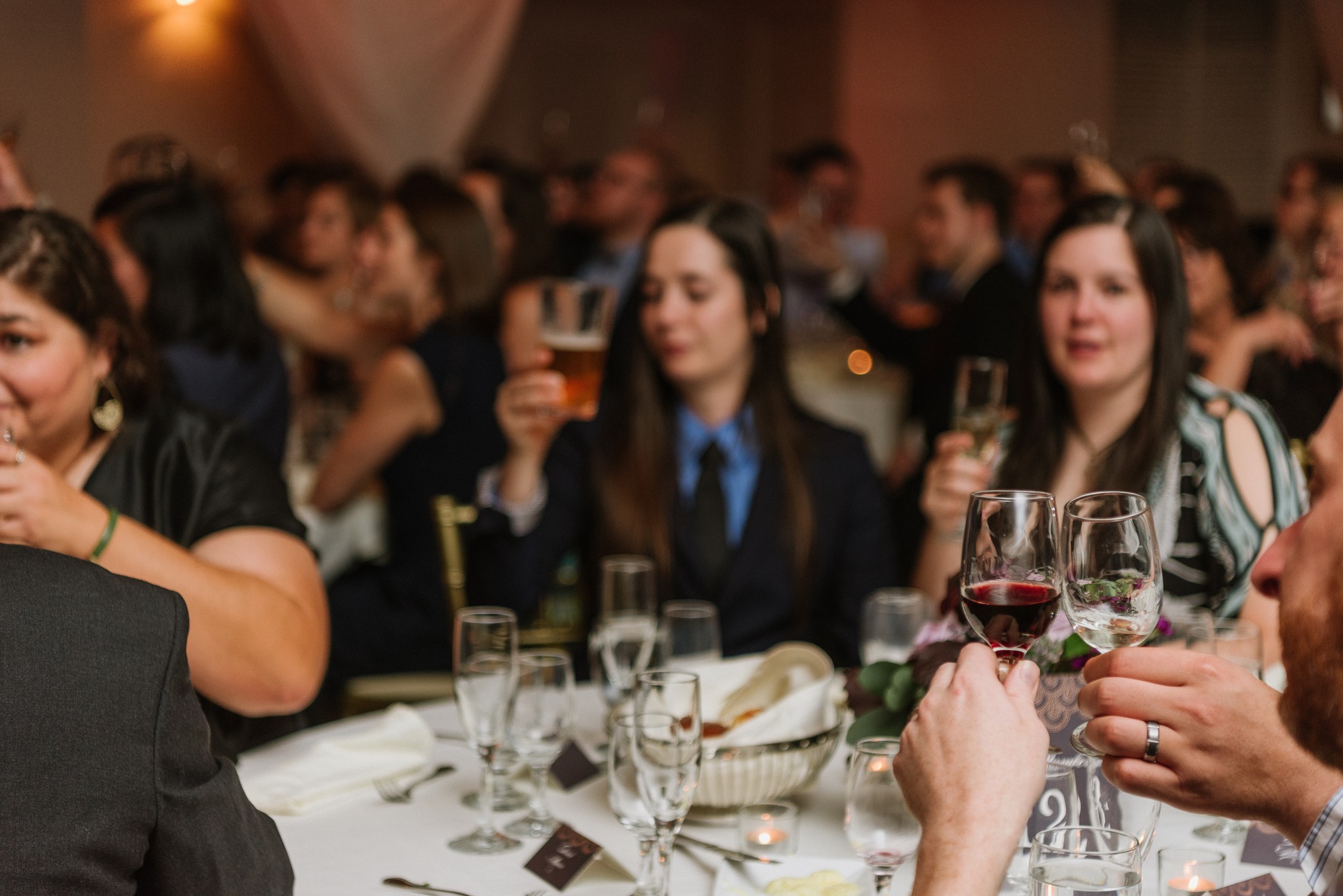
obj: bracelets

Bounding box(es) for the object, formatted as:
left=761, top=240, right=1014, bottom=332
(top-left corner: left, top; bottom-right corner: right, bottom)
left=81, top=499, right=120, bottom=570
left=926, top=520, right=975, bottom=542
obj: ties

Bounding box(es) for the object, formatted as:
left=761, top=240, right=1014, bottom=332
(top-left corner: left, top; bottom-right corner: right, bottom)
left=691, top=440, right=730, bottom=603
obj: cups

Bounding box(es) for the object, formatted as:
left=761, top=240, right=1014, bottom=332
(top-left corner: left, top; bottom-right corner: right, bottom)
left=861, top=586, right=926, bottom=665
left=1152, top=604, right=1263, bottom=681
left=737, top=801, right=796, bottom=858
left=663, top=600, right=722, bottom=723
left=952, top=357, right=1006, bottom=458
left=1158, top=847, right=1226, bottom=896
left=602, top=556, right=656, bottom=703
left=537, top=278, right=619, bottom=422
left=999, top=757, right=1162, bottom=896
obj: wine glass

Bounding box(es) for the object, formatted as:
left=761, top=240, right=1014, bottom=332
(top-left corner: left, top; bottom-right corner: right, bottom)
left=959, top=489, right=1061, bottom=685
left=846, top=737, right=924, bottom=896
left=448, top=605, right=569, bottom=854
left=608, top=670, right=702, bottom=896
left=1061, top=490, right=1164, bottom=756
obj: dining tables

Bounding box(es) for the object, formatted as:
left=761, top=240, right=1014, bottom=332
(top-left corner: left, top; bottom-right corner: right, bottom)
left=229, top=635, right=1317, bottom=895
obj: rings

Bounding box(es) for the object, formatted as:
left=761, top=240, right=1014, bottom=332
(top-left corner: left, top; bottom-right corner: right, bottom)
left=907, top=708, right=921, bottom=722
left=13, top=447, right=26, bottom=468
left=1142, top=720, right=1159, bottom=764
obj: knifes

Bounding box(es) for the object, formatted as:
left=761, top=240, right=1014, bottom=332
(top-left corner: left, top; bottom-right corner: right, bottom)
left=674, top=833, right=784, bottom=866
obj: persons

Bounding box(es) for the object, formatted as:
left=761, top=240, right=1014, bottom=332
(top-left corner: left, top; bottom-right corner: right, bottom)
left=455, top=190, right=909, bottom=681
left=0, top=138, right=337, bottom=752
left=0, top=205, right=341, bottom=764
left=0, top=83, right=1342, bottom=491
left=300, top=173, right=556, bottom=705
left=0, top=538, right=300, bottom=896
left=1064, top=384, right=1343, bottom=896
left=910, top=187, right=1312, bottom=682
left=893, top=641, right=1053, bottom=894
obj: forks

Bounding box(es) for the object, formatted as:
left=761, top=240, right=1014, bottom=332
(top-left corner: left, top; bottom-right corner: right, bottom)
left=374, top=764, right=452, bottom=804
